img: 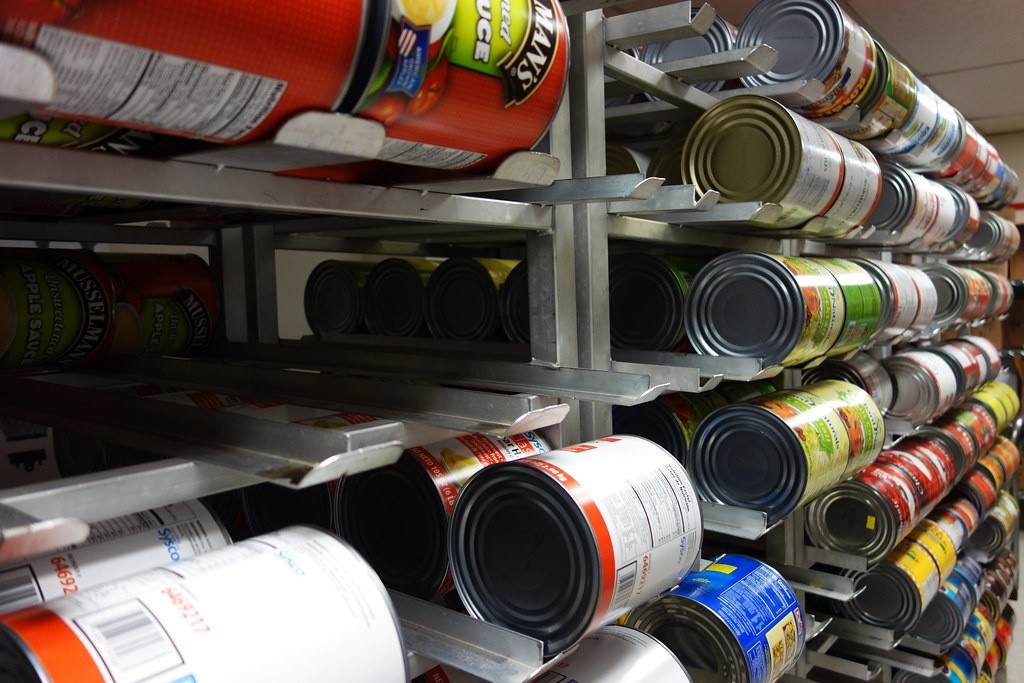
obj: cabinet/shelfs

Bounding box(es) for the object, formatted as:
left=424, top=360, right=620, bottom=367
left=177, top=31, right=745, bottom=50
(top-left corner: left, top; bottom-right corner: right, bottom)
left=1, top=1, right=1023, bottom=683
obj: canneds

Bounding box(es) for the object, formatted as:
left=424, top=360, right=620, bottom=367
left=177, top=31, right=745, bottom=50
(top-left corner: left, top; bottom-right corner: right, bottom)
left=0, top=0, right=1024, bottom=683
